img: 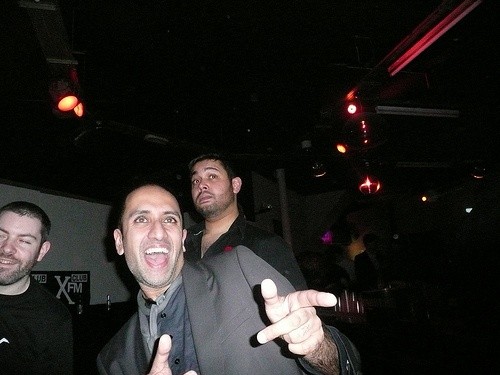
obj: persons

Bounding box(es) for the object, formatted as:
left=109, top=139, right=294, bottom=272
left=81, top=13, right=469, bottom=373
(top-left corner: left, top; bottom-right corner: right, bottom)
left=95, top=183, right=365, bottom=375
left=181, top=154, right=308, bottom=291
left=0, top=201, right=75, bottom=375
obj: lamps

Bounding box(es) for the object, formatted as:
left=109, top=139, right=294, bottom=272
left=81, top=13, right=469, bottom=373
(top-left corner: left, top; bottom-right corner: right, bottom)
left=48, top=77, right=84, bottom=120
left=301, top=140, right=328, bottom=177
